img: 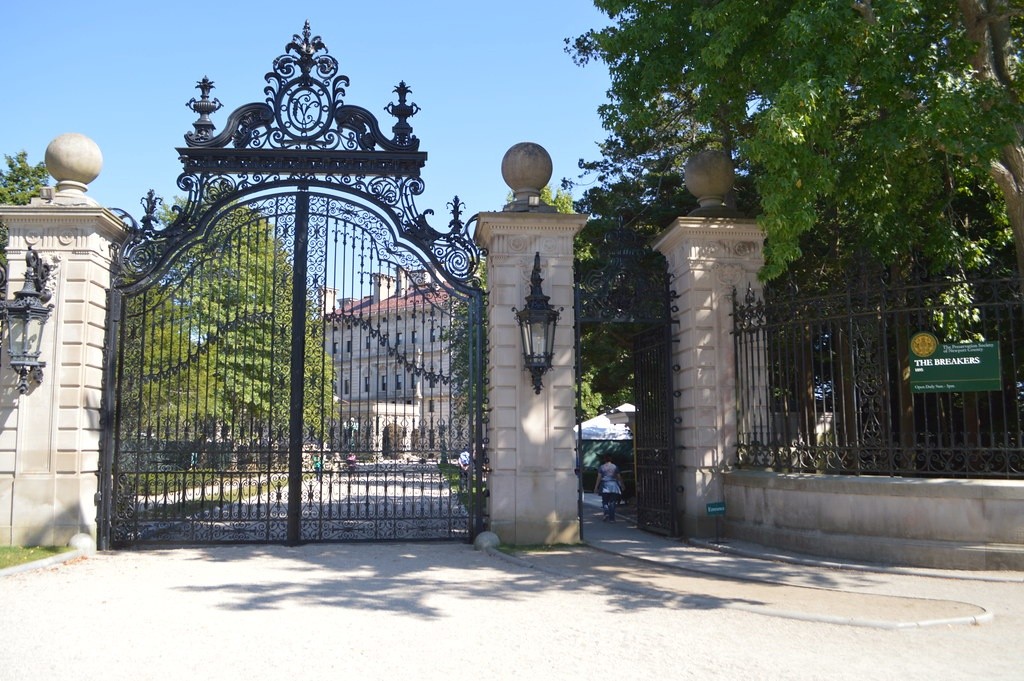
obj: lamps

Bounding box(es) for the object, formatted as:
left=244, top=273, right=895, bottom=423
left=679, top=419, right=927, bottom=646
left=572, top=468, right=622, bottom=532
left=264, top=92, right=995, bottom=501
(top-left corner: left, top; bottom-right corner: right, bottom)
left=511, top=252, right=560, bottom=396
left=0, top=246, right=56, bottom=395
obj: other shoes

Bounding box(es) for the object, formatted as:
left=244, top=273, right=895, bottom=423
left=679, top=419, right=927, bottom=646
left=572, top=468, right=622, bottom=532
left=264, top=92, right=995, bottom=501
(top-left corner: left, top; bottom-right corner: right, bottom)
left=465, top=489, right=469, bottom=493
left=459, top=489, right=464, bottom=493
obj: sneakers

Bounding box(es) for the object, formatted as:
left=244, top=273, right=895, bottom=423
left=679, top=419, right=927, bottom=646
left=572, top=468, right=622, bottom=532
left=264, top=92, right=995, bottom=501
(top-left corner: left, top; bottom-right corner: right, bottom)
left=603, top=513, right=616, bottom=523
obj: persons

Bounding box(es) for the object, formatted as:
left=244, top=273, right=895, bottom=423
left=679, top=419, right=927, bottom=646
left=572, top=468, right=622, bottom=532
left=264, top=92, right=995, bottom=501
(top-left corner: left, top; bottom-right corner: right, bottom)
left=314, top=451, right=356, bottom=482
left=459, top=447, right=471, bottom=491
left=593, top=455, right=627, bottom=524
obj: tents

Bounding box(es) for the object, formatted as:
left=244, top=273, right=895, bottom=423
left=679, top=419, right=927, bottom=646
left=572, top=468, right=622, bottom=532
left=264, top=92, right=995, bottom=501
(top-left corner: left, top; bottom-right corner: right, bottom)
left=573, top=403, right=638, bottom=494
